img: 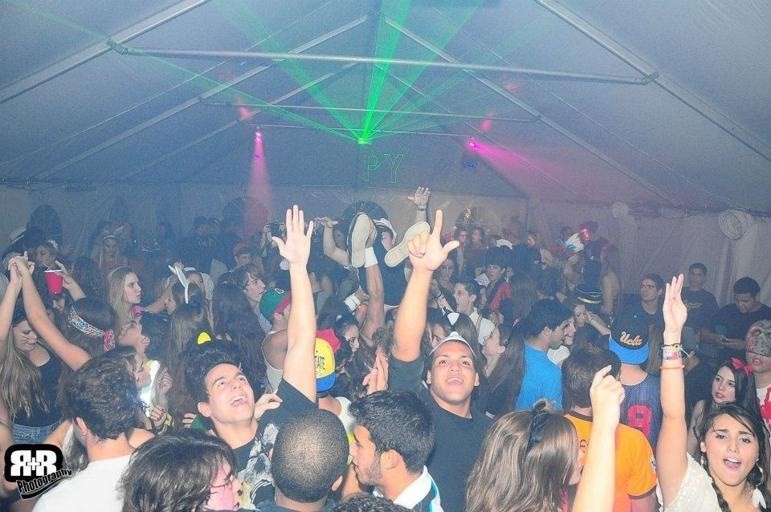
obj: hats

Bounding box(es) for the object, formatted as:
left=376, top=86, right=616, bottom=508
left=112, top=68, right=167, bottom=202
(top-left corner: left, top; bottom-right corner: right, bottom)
left=609, top=314, right=649, bottom=364
left=259, top=288, right=291, bottom=319
left=314, top=338, right=336, bottom=391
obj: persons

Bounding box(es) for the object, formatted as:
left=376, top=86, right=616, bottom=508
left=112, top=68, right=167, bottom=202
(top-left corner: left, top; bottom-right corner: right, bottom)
left=0, top=186, right=770, bottom=512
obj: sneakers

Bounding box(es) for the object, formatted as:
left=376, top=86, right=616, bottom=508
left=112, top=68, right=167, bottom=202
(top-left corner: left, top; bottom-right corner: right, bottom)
left=347, top=212, right=370, bottom=268
left=384, top=222, right=430, bottom=268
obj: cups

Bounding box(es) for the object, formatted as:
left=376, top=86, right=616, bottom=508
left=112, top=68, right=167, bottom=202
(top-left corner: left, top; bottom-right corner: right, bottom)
left=44, top=269, right=64, bottom=295
left=579, top=227, right=592, bottom=244
